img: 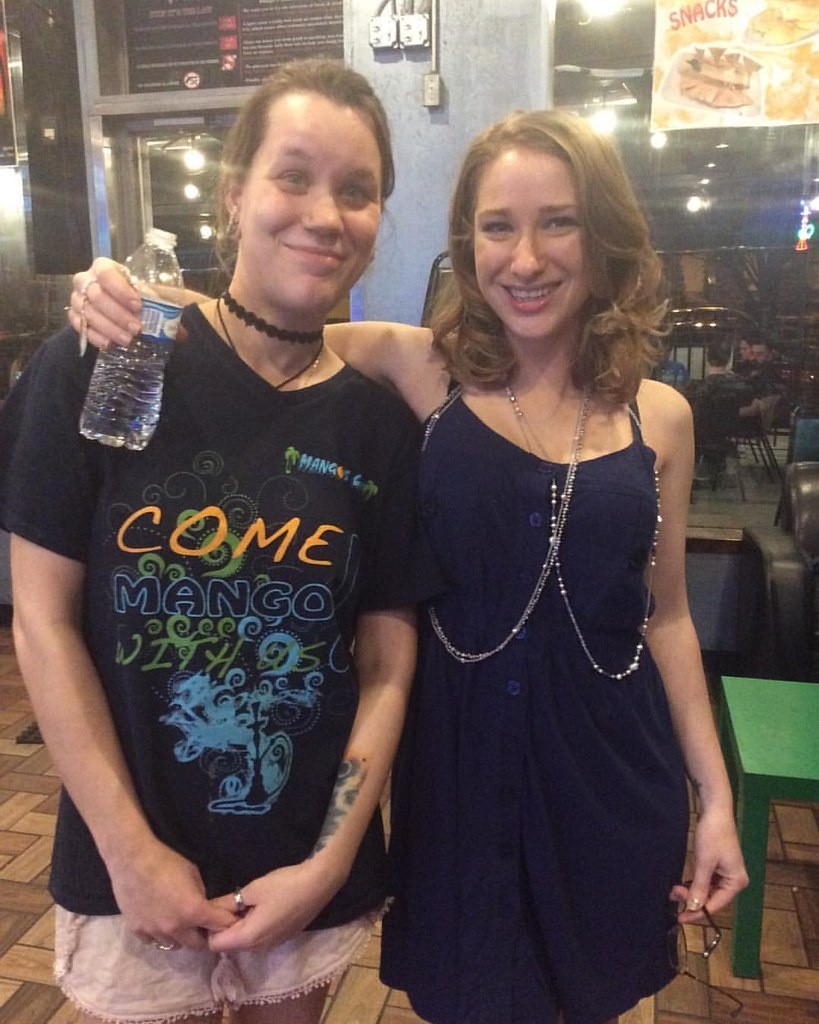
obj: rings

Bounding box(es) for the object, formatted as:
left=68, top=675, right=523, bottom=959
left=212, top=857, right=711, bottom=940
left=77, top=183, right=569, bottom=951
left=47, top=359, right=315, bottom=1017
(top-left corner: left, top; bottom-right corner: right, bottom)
left=83, top=278, right=100, bottom=295
left=153, top=941, right=175, bottom=951
left=232, top=890, right=244, bottom=909
left=74, top=296, right=87, bottom=310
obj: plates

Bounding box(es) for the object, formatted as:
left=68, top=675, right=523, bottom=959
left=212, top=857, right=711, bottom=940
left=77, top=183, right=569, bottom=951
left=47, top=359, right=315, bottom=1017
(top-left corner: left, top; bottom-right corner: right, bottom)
left=742, top=0, right=819, bottom=49
left=657, top=45, right=765, bottom=120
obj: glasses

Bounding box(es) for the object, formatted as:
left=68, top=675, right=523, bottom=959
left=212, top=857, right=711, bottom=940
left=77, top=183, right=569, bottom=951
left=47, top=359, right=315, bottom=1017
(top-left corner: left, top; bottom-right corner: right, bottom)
left=668, top=880, right=744, bottom=1015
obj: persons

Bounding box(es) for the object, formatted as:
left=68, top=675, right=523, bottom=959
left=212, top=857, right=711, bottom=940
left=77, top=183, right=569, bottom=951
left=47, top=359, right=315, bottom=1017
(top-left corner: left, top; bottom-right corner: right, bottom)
left=682, top=337, right=751, bottom=468
left=739, top=333, right=784, bottom=439
left=66, top=113, right=748, bottom=1023
left=0, top=58, right=427, bottom=1024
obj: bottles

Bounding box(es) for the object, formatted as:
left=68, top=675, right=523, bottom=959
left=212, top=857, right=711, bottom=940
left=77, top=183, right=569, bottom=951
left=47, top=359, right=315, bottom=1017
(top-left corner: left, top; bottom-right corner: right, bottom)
left=77, top=228, right=184, bottom=451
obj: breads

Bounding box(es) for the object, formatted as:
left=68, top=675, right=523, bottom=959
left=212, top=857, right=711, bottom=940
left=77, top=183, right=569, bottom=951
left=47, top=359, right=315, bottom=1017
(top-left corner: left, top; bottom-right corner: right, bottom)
left=679, top=0, right=819, bottom=121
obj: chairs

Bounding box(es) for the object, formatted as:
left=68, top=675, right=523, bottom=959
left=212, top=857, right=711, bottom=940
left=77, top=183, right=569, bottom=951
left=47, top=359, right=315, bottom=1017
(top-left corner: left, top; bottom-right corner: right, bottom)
left=689, top=400, right=780, bottom=505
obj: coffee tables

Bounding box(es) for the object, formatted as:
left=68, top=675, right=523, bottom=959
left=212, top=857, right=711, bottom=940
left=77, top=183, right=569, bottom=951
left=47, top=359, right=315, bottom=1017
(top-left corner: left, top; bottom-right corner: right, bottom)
left=716, top=676, right=819, bottom=979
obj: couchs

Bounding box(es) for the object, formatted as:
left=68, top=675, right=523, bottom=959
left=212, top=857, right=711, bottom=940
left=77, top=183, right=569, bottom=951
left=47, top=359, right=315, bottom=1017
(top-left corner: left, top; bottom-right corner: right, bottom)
left=736, top=461, right=819, bottom=684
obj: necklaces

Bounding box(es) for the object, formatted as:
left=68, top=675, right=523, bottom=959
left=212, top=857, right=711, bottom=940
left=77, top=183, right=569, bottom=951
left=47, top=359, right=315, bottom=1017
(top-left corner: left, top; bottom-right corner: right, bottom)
left=218, top=300, right=322, bottom=389
left=420, top=361, right=662, bottom=679
left=221, top=293, right=323, bottom=344
